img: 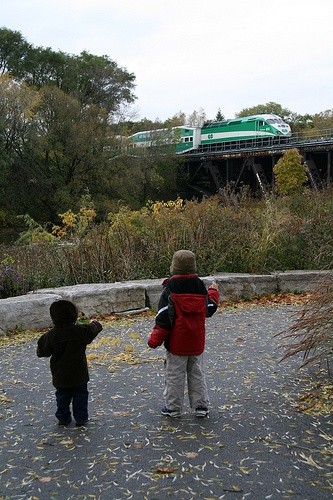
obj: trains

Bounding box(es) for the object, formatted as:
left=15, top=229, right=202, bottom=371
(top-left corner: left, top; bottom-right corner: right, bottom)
left=101, top=113, right=293, bottom=159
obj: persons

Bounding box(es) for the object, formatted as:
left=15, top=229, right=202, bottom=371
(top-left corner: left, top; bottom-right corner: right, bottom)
left=37, top=300, right=103, bottom=426
left=147, top=251, right=219, bottom=418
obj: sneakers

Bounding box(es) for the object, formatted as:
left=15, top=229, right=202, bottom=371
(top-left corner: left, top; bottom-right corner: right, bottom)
left=195, top=405, right=209, bottom=416
left=161, top=406, right=181, bottom=417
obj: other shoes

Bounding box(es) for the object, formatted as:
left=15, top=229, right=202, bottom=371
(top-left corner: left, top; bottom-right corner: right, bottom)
left=75, top=419, right=88, bottom=426
left=56, top=419, right=69, bottom=425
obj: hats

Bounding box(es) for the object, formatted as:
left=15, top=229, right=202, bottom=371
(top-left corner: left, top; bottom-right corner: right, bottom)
left=170, top=250, right=197, bottom=275
